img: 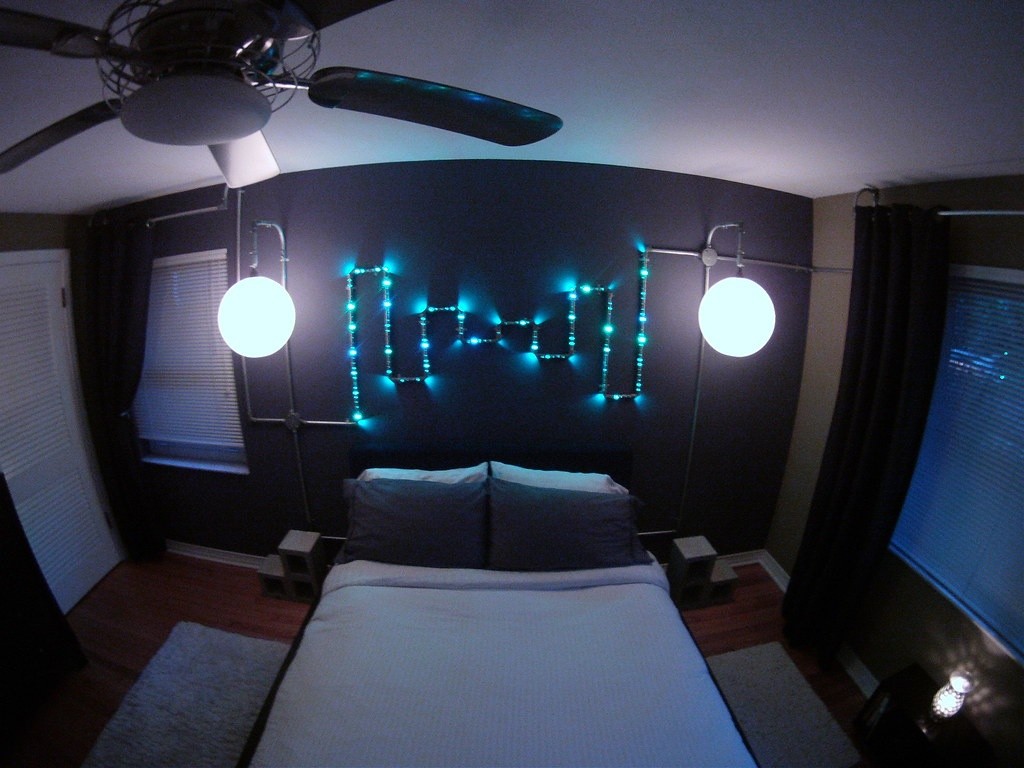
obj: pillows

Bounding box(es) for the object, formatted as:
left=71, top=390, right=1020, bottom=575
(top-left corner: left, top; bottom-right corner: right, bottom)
left=360, top=460, right=487, bottom=485
left=489, top=479, right=654, bottom=572
left=336, top=476, right=492, bottom=566
left=490, top=462, right=630, bottom=497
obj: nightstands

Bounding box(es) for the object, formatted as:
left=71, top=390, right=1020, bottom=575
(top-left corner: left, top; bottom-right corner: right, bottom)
left=667, top=535, right=718, bottom=603
left=275, top=529, right=327, bottom=603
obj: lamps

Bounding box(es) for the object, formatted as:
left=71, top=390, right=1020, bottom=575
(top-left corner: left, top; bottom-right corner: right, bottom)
left=679, top=218, right=777, bottom=528
left=917, top=661, right=983, bottom=740
left=93, top=0, right=320, bottom=148
left=218, top=222, right=313, bottom=528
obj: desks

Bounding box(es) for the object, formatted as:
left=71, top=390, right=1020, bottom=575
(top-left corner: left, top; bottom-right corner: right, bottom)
left=849, top=661, right=1007, bottom=768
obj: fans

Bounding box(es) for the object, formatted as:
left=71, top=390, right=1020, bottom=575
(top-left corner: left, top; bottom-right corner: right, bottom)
left=0, top=0, right=565, bottom=189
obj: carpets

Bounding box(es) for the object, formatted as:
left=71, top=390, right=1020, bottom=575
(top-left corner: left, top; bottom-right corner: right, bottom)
left=706, top=636, right=866, bottom=768
left=81, top=621, right=283, bottom=768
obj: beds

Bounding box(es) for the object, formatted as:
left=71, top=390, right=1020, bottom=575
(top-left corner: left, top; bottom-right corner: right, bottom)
left=240, top=459, right=763, bottom=768
